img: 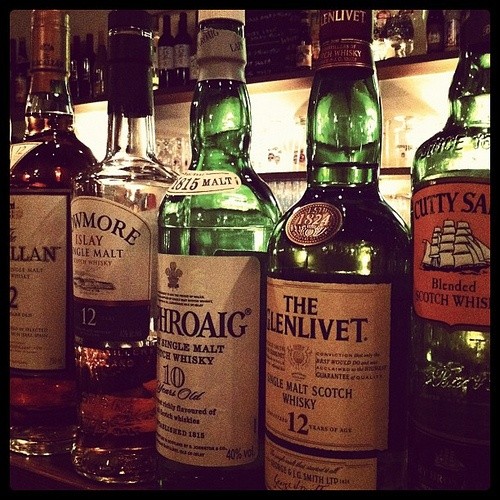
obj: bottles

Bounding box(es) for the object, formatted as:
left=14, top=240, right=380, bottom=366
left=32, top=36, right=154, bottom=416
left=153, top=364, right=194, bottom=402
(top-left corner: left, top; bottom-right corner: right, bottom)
left=80, top=33, right=95, bottom=102
left=175, top=12, right=192, bottom=89
left=443, top=10, right=461, bottom=53
left=8, top=8, right=98, bottom=458
left=426, top=9, right=443, bottom=53
left=158, top=14, right=175, bottom=90
left=92, top=31, right=108, bottom=99
left=67, top=8, right=179, bottom=487
left=68, top=34, right=82, bottom=103
left=260, top=8, right=410, bottom=494
left=393, top=8, right=490, bottom=494
left=13, top=37, right=31, bottom=106
left=155, top=8, right=282, bottom=491
left=152, top=13, right=160, bottom=90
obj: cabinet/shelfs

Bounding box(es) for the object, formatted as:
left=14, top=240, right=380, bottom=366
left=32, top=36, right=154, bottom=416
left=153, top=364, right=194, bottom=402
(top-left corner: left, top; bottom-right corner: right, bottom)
left=11, top=48, right=490, bottom=185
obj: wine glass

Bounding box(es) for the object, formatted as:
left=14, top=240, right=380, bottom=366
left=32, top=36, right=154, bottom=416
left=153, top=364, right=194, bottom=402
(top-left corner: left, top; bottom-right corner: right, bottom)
left=156, top=135, right=191, bottom=173
